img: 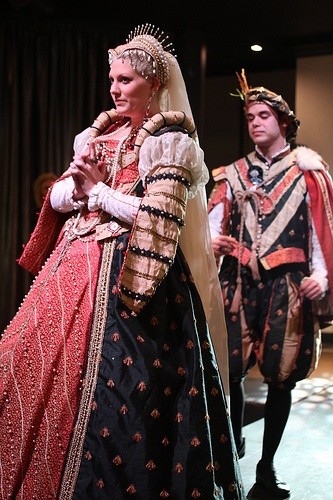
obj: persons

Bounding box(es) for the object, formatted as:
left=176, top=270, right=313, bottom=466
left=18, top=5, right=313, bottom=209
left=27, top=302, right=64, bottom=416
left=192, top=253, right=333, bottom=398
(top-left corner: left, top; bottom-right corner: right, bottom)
left=0, top=23, right=246, bottom=500
left=206, top=69, right=333, bottom=500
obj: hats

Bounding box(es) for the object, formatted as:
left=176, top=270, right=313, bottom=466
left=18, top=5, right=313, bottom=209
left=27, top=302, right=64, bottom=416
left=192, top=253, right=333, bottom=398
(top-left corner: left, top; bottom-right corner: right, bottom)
left=231, top=69, right=300, bottom=140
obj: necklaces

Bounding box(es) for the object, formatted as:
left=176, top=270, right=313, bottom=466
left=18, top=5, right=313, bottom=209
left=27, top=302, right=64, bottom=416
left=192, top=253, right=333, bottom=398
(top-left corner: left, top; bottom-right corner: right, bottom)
left=73, top=116, right=132, bottom=243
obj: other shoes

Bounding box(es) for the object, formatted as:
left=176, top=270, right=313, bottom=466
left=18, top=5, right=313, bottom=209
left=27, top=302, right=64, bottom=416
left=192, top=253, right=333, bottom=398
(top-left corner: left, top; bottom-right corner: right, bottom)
left=256, top=459, right=279, bottom=499
left=238, top=437, right=246, bottom=458
